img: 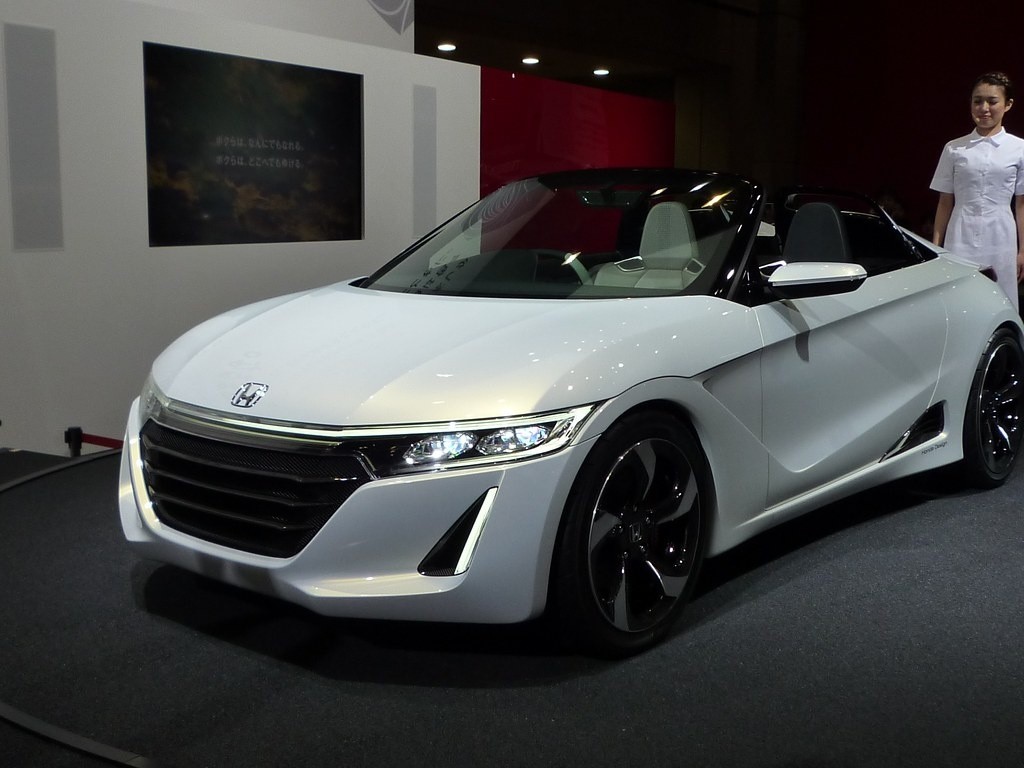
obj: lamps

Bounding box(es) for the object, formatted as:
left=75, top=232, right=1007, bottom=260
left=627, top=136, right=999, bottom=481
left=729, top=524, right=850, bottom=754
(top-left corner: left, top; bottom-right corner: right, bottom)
left=521, top=54, right=539, bottom=65
left=593, top=65, right=610, bottom=76
left=435, top=39, right=457, bottom=55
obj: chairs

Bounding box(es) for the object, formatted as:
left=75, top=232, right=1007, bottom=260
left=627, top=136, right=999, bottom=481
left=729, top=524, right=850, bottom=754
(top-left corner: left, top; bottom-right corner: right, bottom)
left=784, top=202, right=852, bottom=267
left=595, top=201, right=705, bottom=288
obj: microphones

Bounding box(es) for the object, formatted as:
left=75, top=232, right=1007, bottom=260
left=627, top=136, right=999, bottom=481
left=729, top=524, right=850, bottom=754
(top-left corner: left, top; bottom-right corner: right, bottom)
left=972, top=113, right=980, bottom=123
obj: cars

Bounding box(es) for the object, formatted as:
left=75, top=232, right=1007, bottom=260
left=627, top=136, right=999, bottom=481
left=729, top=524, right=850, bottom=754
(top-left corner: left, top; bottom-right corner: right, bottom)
left=117, top=162, right=1024, bottom=654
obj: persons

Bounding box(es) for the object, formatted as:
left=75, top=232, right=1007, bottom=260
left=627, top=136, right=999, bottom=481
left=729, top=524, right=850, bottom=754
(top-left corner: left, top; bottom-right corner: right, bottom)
left=928, top=72, right=1024, bottom=319
left=891, top=200, right=910, bottom=230
